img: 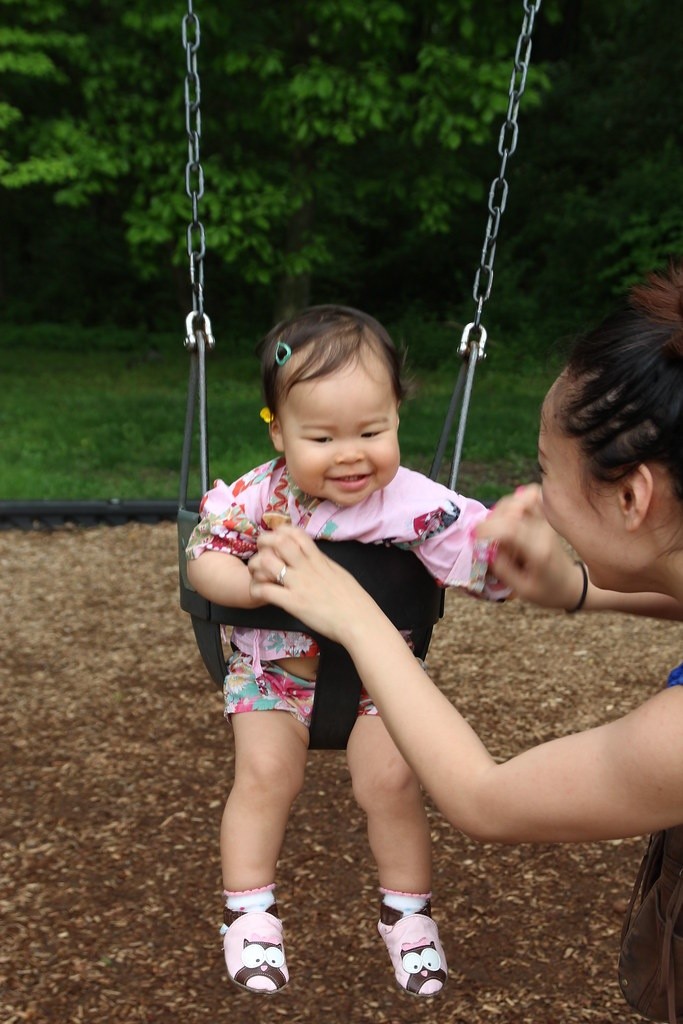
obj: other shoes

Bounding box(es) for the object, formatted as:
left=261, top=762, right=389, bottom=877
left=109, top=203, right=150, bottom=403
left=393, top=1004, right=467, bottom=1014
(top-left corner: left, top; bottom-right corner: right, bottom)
left=377, top=898, right=449, bottom=997
left=220, top=903, right=290, bottom=993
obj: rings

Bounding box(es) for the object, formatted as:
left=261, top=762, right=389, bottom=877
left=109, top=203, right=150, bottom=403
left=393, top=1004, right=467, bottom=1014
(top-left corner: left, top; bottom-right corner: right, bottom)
left=276, top=566, right=287, bottom=583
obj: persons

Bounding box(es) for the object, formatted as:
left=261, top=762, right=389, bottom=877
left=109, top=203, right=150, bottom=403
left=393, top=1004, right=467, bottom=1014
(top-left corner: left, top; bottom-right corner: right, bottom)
left=184, top=301, right=551, bottom=999
left=246, top=270, right=683, bottom=1024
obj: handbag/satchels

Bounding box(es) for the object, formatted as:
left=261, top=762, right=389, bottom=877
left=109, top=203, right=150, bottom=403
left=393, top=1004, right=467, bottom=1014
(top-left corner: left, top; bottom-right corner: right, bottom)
left=618, top=824, right=683, bottom=1024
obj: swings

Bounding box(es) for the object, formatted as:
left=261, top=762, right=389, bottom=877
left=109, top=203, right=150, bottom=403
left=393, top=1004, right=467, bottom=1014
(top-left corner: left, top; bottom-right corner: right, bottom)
left=172, top=0, right=550, bottom=753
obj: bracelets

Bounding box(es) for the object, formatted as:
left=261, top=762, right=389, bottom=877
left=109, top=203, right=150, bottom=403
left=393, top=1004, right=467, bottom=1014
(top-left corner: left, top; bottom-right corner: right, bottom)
left=565, top=559, right=587, bottom=615
